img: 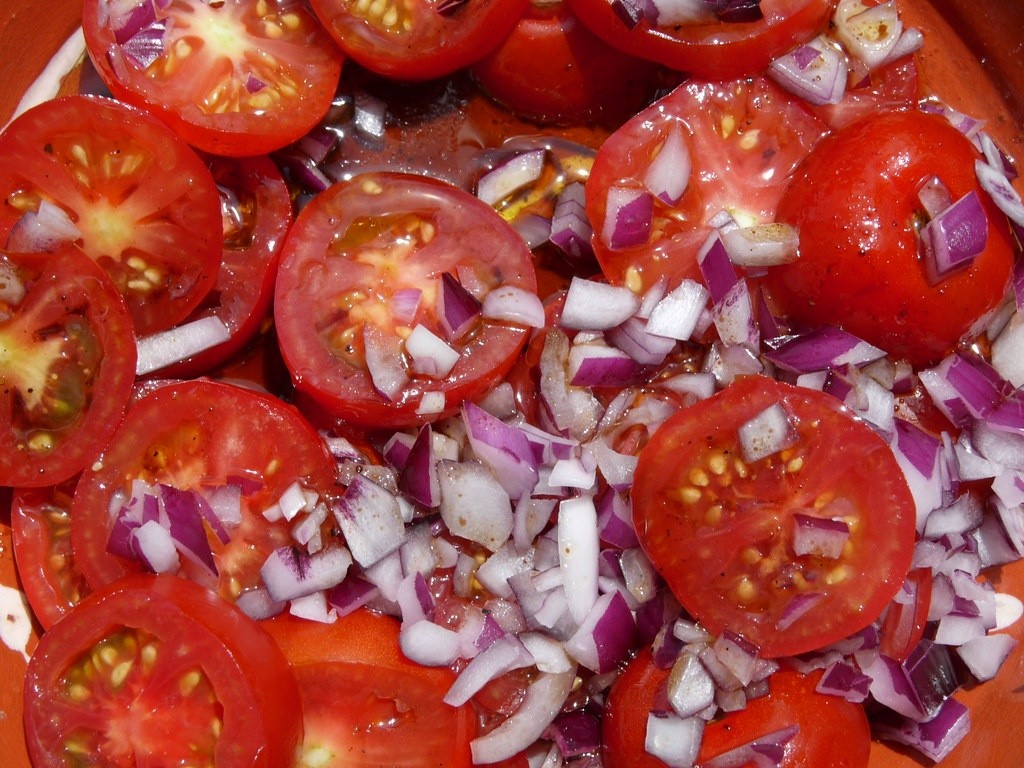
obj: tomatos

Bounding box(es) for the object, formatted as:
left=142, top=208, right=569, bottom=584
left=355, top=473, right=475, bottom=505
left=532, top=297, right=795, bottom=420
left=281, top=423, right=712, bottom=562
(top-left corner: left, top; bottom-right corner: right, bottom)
left=1, top=0, right=1009, bottom=768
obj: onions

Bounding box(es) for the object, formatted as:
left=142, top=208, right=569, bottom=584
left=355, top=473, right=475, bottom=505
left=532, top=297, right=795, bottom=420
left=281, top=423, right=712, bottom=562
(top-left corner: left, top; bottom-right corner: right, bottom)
left=11, top=0, right=1024, bottom=767
